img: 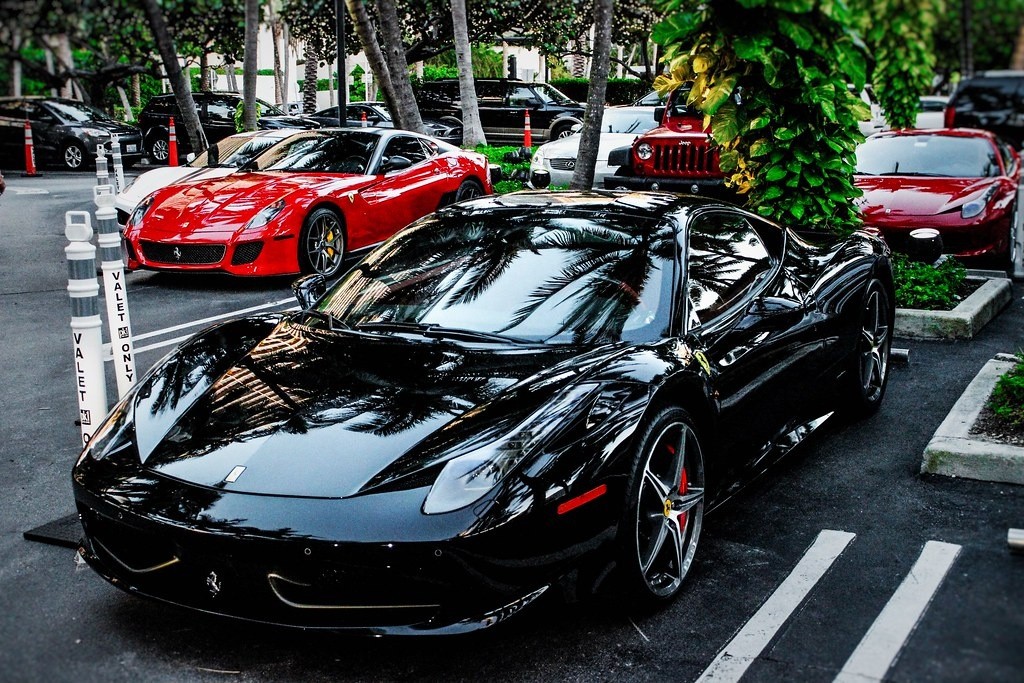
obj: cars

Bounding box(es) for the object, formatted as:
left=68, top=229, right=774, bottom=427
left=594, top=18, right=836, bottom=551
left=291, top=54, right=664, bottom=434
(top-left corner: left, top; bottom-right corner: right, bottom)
left=611, top=82, right=745, bottom=113
left=910, top=95, right=953, bottom=130
left=0, top=96, right=147, bottom=173
left=304, top=98, right=463, bottom=142
left=267, top=100, right=306, bottom=118
left=526, top=105, right=684, bottom=189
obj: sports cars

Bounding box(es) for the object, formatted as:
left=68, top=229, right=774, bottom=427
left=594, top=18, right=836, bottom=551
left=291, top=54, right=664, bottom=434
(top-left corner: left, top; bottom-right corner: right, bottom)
left=111, top=129, right=312, bottom=240
left=120, top=127, right=494, bottom=283
left=71, top=188, right=898, bottom=641
left=845, top=128, right=1023, bottom=279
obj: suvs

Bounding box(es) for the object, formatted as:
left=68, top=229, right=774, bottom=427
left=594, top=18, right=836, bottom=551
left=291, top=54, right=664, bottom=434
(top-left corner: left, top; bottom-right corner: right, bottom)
left=841, top=83, right=891, bottom=139
left=601, top=77, right=770, bottom=207
left=413, top=75, right=585, bottom=145
left=946, top=69, right=1024, bottom=155
left=134, top=91, right=324, bottom=165
